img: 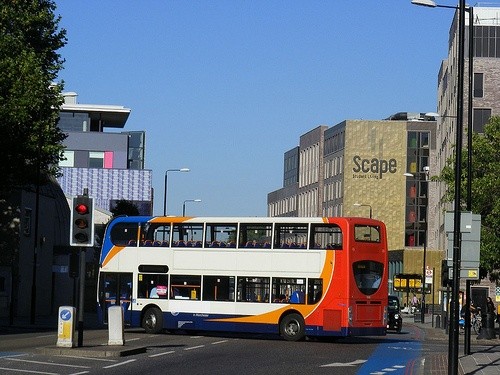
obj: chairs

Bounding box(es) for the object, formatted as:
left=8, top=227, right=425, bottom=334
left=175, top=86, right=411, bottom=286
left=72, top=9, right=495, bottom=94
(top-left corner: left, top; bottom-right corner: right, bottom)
left=128, top=239, right=307, bottom=249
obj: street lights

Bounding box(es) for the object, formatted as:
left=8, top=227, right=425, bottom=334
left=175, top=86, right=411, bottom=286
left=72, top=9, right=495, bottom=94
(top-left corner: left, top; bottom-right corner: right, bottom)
left=421, top=111, right=463, bottom=340
left=163, top=168, right=191, bottom=216
left=403, top=173, right=427, bottom=324
left=182, top=198, right=203, bottom=216
left=353, top=202, right=373, bottom=219
left=412, top=1, right=474, bottom=355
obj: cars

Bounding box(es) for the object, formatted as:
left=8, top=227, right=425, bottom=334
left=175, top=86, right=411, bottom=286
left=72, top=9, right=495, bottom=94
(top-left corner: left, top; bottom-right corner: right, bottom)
left=388, top=294, right=403, bottom=333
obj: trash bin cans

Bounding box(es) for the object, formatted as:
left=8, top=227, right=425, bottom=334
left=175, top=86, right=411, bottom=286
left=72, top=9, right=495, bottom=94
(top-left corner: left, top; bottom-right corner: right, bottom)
left=414, top=309, right=421, bottom=323
left=108, top=306, right=125, bottom=346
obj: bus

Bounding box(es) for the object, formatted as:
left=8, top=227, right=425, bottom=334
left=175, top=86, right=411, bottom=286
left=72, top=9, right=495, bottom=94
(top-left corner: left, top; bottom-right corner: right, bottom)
left=95, top=216, right=388, bottom=340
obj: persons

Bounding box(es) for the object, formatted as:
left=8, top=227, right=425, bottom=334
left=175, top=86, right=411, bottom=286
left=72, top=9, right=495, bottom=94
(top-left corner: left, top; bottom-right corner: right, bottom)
left=481, top=297, right=496, bottom=327
left=411, top=295, right=421, bottom=314
left=460, top=299, right=480, bottom=327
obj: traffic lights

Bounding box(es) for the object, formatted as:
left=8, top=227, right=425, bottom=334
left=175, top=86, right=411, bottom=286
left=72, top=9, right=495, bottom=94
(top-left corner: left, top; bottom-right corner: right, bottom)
left=70, top=195, right=94, bottom=247
